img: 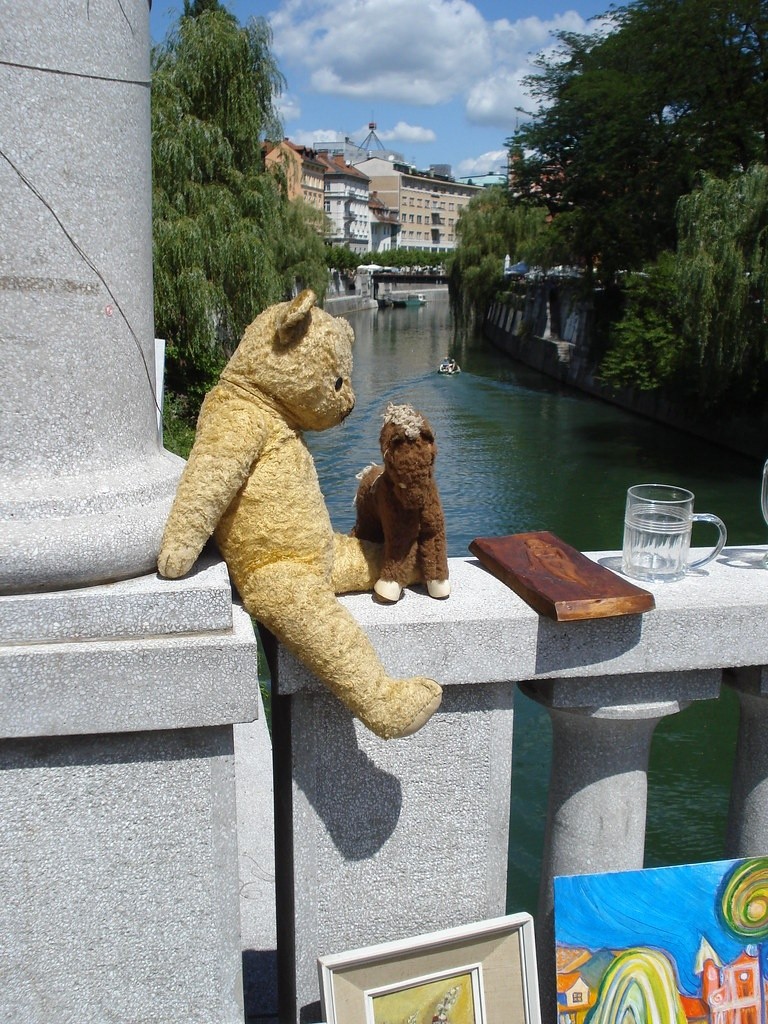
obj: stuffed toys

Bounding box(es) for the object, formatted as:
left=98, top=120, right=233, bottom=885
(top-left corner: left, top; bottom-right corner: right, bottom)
left=158, top=289, right=443, bottom=740
left=352, top=400, right=451, bottom=601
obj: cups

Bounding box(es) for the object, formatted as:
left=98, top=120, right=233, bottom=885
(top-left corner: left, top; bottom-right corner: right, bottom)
left=620, top=484, right=728, bottom=583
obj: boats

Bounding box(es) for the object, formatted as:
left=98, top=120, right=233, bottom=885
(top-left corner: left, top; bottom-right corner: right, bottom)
left=374, top=288, right=395, bottom=308
left=388, top=293, right=428, bottom=307
left=439, top=363, right=462, bottom=377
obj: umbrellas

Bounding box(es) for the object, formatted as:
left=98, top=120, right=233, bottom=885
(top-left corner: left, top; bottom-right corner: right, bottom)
left=357, top=264, right=382, bottom=275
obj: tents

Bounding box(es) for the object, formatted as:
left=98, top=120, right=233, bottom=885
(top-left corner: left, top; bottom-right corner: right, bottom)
left=506, top=262, right=529, bottom=272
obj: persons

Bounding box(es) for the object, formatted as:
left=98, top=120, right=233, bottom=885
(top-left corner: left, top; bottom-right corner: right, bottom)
left=525, top=539, right=585, bottom=583
